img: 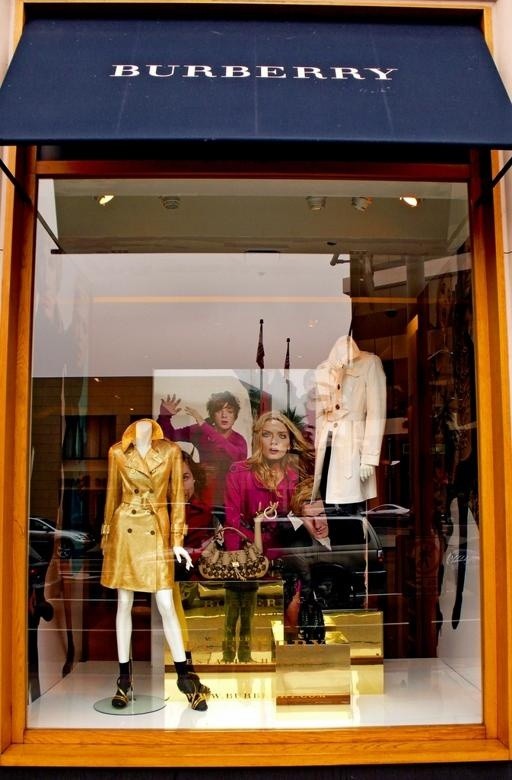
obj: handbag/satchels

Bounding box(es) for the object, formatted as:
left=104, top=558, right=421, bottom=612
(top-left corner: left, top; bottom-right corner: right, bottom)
left=197, top=541, right=270, bottom=580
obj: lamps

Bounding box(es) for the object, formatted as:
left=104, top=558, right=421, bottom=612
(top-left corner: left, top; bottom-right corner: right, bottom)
left=303, top=194, right=372, bottom=216
left=91, top=188, right=180, bottom=213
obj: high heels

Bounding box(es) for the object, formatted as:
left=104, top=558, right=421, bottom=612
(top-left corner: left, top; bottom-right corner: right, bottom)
left=177, top=679, right=207, bottom=710
left=186, top=659, right=211, bottom=694
left=63, top=644, right=74, bottom=674
left=112, top=677, right=132, bottom=708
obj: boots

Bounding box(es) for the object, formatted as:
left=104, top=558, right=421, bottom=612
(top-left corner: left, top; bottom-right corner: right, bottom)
left=298, top=589, right=325, bottom=644
left=238, top=646, right=257, bottom=662
left=221, top=640, right=236, bottom=662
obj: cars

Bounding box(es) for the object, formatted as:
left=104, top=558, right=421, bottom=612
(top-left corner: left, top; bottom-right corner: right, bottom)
left=28, top=503, right=413, bottom=641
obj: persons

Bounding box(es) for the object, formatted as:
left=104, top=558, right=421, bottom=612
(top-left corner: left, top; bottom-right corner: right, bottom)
left=167, top=450, right=213, bottom=581
left=221, top=583, right=259, bottom=661
left=100, top=419, right=208, bottom=710
left=224, top=411, right=315, bottom=643
left=309, top=336, right=387, bottom=608
left=156, top=392, right=248, bottom=506
left=54, top=487, right=89, bottom=676
left=435, top=461, right=470, bottom=629
left=280, top=478, right=384, bottom=644
left=28, top=585, right=53, bottom=703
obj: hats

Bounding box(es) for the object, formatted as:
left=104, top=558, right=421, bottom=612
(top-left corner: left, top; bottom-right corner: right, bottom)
left=173, top=441, right=201, bottom=464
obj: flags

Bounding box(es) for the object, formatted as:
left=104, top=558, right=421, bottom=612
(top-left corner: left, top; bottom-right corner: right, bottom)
left=256, top=324, right=265, bottom=369
left=284, top=343, right=289, bottom=378
